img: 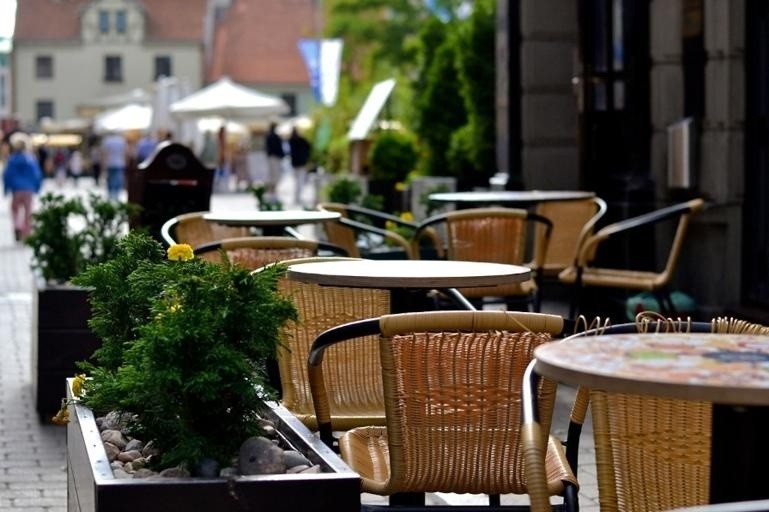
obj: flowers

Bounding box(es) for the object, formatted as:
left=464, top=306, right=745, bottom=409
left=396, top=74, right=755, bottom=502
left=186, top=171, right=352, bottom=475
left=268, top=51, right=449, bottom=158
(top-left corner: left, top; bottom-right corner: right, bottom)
left=64, top=233, right=301, bottom=474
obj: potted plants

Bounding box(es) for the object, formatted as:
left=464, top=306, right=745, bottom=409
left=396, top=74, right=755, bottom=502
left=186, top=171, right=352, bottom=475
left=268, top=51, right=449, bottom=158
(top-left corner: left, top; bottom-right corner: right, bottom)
left=26, top=190, right=156, bottom=426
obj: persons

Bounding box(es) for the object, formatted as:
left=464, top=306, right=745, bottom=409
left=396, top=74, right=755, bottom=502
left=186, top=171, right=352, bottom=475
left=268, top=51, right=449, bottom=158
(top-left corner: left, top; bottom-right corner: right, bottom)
left=2, top=130, right=43, bottom=241
left=38, top=143, right=84, bottom=189
left=90, top=116, right=316, bottom=210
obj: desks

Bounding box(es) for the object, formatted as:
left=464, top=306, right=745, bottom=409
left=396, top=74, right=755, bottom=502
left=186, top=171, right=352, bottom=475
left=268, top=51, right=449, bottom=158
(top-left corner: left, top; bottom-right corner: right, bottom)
left=534, top=335, right=769, bottom=512
left=201, top=209, right=343, bottom=248
left=431, top=188, right=595, bottom=315
left=286, top=255, right=531, bottom=508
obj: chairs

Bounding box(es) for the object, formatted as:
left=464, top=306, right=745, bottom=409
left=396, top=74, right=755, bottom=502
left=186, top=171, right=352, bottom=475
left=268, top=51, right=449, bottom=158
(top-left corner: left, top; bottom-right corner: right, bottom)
left=519, top=309, right=769, bottom=512
left=308, top=310, right=591, bottom=512
left=157, top=209, right=476, bottom=506
left=316, top=193, right=706, bottom=332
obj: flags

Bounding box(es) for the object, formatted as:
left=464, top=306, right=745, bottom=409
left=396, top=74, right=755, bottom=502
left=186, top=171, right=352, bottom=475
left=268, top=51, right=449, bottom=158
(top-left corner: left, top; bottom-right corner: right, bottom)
left=297, top=35, right=345, bottom=105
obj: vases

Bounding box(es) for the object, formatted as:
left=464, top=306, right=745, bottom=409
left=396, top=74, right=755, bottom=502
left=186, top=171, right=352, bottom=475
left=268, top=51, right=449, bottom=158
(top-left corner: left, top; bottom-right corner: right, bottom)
left=64, top=360, right=362, bottom=512
